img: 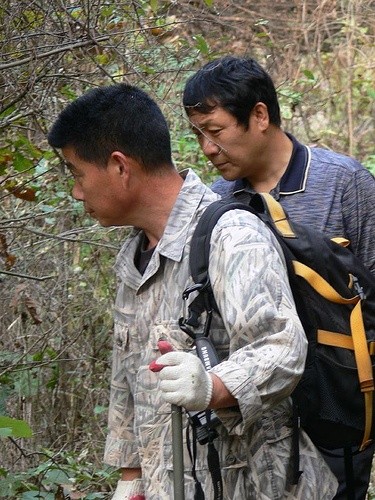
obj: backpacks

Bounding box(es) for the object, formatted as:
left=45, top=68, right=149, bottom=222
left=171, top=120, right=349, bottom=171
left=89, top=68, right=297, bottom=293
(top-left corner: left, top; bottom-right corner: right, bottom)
left=184, top=191, right=375, bottom=487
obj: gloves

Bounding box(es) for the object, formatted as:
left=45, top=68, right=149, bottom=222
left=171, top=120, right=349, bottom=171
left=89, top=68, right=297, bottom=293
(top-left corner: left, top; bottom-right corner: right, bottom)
left=111, top=478, right=144, bottom=500
left=150, top=340, right=212, bottom=411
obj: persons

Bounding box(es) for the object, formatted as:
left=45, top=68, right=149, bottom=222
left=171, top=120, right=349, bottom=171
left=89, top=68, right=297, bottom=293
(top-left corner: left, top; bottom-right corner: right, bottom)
left=183, top=55, right=375, bottom=500
left=48, top=85, right=340, bottom=500
